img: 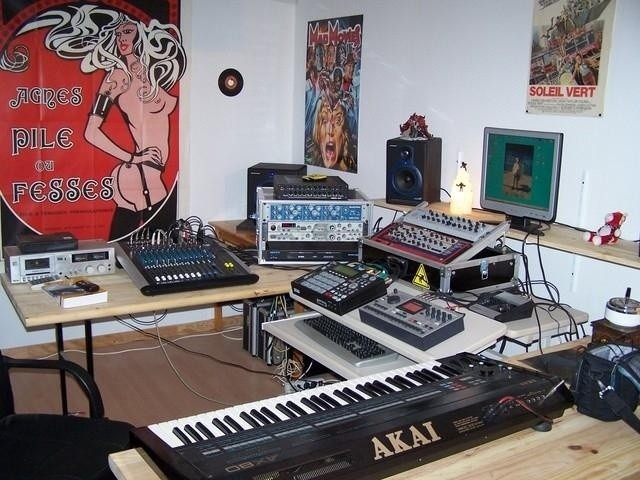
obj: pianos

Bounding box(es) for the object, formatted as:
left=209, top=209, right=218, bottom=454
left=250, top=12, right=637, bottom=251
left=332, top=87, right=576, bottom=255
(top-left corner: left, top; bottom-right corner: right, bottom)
left=129, top=351, right=574, bottom=480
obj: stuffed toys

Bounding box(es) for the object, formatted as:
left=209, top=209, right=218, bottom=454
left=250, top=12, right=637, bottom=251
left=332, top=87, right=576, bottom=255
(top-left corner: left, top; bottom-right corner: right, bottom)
left=582, top=211, right=626, bottom=247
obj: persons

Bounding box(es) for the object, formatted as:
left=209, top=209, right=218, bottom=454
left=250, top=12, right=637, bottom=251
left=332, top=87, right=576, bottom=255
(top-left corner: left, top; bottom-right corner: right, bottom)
left=84, top=12, right=178, bottom=242
left=305, top=42, right=361, bottom=173
left=511, top=157, right=521, bottom=190
left=569, top=54, right=599, bottom=85
left=560, top=4, right=576, bottom=32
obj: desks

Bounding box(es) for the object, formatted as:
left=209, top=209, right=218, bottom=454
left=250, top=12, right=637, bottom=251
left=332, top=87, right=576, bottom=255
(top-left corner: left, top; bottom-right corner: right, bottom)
left=0, top=249, right=310, bottom=418
left=372, top=198, right=640, bottom=270
left=109, top=337, right=640, bottom=480
left=301, top=234, right=589, bottom=346
left=209, top=220, right=322, bottom=377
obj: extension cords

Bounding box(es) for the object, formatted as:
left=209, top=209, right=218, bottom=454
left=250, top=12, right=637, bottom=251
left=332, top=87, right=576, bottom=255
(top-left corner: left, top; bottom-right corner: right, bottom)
left=284, top=378, right=324, bottom=394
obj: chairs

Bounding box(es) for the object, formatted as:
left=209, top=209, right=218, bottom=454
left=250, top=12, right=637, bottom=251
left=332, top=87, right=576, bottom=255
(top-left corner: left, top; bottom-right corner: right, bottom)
left=0, top=348, right=137, bottom=480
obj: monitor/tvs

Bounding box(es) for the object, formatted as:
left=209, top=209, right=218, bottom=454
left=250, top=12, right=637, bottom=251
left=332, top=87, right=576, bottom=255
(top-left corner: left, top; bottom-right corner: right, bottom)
left=478, top=128, right=563, bottom=236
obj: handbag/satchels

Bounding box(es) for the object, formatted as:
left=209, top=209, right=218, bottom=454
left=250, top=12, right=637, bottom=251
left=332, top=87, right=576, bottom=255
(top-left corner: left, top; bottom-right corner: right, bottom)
left=575, top=341, right=640, bottom=422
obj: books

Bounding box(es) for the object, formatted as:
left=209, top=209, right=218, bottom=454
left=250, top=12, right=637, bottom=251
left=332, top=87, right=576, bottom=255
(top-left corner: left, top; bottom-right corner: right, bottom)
left=41, top=276, right=107, bottom=309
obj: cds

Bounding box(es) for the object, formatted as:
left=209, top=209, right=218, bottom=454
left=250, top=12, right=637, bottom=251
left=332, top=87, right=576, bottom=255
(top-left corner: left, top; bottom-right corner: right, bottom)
left=607, top=297, right=640, bottom=314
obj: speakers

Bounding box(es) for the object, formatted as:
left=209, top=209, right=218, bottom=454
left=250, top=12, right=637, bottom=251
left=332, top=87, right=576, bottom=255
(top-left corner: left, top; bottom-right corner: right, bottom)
left=386, top=136, right=441, bottom=206
left=247, top=163, right=307, bottom=220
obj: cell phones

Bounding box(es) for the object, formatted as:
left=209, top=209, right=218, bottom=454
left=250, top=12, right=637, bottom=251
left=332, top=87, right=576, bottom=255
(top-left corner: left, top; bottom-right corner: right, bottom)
left=76, top=280, right=99, bottom=292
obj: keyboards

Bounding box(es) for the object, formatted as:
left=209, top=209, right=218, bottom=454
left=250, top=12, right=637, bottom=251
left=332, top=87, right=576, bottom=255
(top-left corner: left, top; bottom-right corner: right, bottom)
left=294, top=315, right=398, bottom=368
